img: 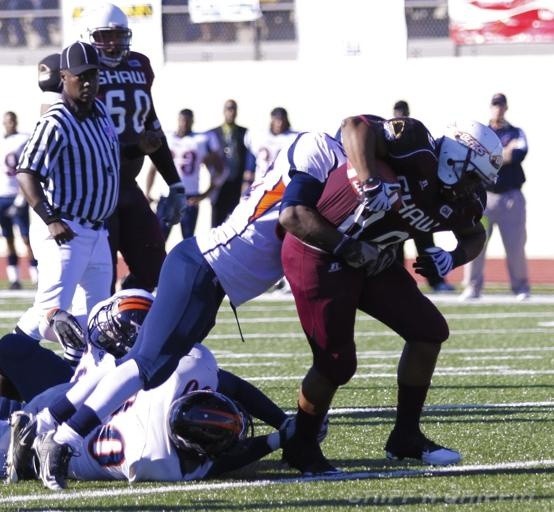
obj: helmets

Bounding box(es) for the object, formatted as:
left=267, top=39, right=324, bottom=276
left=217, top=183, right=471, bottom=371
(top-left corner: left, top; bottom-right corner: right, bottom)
left=87, top=288, right=155, bottom=360
left=78, top=2, right=133, bottom=68
left=379, top=117, right=438, bottom=194
left=437, top=120, right=505, bottom=204
left=167, top=389, right=254, bottom=455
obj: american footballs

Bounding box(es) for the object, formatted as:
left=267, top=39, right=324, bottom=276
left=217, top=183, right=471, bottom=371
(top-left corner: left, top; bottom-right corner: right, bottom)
left=347, top=158, right=401, bottom=209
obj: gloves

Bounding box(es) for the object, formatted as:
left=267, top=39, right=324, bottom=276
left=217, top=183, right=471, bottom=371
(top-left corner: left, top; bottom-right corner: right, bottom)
left=332, top=233, right=382, bottom=273
left=162, top=187, right=188, bottom=227
left=413, top=247, right=454, bottom=281
left=361, top=173, right=402, bottom=212
left=279, top=412, right=329, bottom=448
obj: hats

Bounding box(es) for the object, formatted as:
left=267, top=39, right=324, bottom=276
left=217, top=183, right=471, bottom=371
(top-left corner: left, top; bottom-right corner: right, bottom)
left=492, top=93, right=506, bottom=104
left=59, top=41, right=105, bottom=75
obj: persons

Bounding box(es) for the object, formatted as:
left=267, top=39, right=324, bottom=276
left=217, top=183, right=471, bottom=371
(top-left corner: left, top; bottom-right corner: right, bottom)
left=1, top=4, right=505, bottom=491
left=459, top=93, right=530, bottom=302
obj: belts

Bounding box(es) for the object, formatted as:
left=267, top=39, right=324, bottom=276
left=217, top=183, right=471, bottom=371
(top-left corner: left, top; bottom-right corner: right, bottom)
left=56, top=212, right=102, bottom=231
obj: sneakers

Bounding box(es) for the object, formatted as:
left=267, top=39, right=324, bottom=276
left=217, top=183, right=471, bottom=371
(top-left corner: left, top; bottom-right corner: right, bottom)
left=459, top=287, right=479, bottom=300
left=2, top=410, right=38, bottom=483
left=517, top=292, right=529, bottom=302
left=283, top=438, right=339, bottom=476
left=385, top=430, right=460, bottom=465
left=33, top=421, right=81, bottom=493
left=45, top=308, right=88, bottom=352
